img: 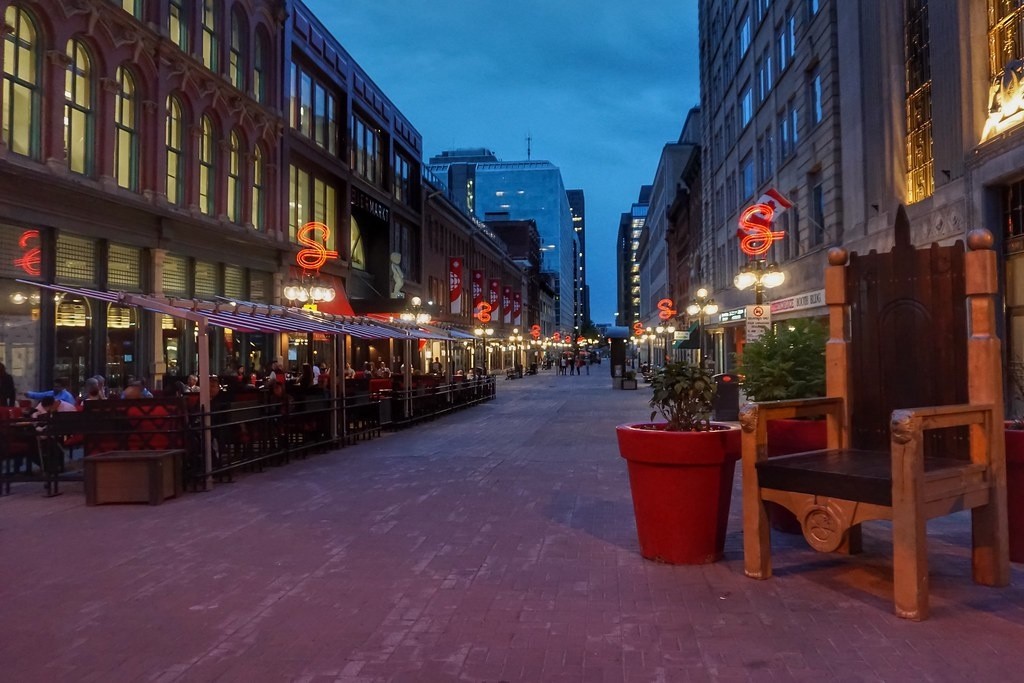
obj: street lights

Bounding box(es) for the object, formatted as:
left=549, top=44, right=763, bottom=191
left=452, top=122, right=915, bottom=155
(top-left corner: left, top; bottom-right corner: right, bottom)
left=655, top=320, right=675, bottom=354
left=284, top=274, right=336, bottom=366
left=474, top=323, right=494, bottom=370
left=399, top=296, right=432, bottom=369
left=640, top=327, right=656, bottom=373
left=686, top=288, right=719, bottom=369
left=531, top=340, right=542, bottom=363
left=633, top=334, right=645, bottom=373
left=508, top=328, right=523, bottom=363
left=734, top=259, right=786, bottom=304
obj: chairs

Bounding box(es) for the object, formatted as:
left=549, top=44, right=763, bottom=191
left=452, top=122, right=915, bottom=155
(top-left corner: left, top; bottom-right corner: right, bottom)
left=738, top=228, right=1011, bottom=622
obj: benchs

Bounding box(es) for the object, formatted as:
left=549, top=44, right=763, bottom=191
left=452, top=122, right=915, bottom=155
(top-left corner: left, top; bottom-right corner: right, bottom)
left=0, top=406, right=32, bottom=474
left=83, top=397, right=199, bottom=488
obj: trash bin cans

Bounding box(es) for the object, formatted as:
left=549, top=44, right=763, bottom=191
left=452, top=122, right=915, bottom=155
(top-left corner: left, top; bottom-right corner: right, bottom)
left=711, top=373, right=739, bottom=422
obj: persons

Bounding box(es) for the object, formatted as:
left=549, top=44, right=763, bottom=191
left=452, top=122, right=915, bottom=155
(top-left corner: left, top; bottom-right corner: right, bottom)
left=1, top=355, right=485, bottom=487
left=507, top=349, right=600, bottom=380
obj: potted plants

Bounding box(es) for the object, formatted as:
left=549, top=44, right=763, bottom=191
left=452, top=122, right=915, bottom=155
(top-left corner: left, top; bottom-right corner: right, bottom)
left=728, top=318, right=830, bottom=533
left=617, top=359, right=743, bottom=565
left=1004, top=355, right=1024, bottom=563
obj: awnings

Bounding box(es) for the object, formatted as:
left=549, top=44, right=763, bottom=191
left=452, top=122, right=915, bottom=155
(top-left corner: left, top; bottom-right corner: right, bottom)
left=670, top=320, right=700, bottom=351
left=15, top=279, right=483, bottom=346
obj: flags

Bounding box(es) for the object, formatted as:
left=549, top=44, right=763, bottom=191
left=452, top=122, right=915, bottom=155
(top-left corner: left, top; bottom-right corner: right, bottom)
left=737, top=189, right=792, bottom=245
